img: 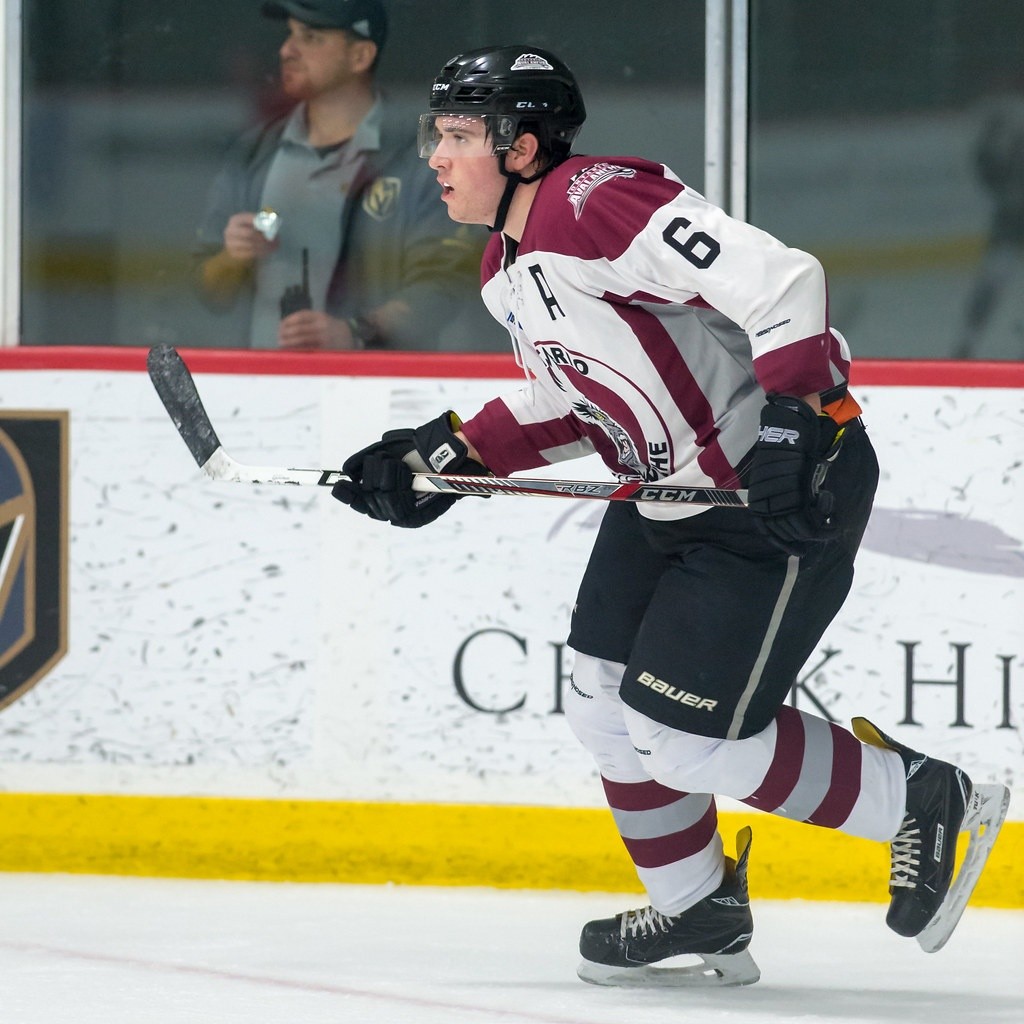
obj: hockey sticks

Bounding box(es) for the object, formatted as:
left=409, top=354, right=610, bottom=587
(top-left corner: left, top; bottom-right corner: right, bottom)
left=146, top=343, right=748, bottom=509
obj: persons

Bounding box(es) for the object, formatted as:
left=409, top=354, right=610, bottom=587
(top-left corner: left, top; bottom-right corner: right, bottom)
left=333, top=45, right=1010, bottom=990
left=188, top=0, right=491, bottom=353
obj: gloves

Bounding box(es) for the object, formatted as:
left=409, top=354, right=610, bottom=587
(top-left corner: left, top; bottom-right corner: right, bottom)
left=332, top=408, right=492, bottom=528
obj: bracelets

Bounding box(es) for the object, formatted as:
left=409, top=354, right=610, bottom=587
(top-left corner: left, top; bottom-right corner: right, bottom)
left=345, top=305, right=389, bottom=351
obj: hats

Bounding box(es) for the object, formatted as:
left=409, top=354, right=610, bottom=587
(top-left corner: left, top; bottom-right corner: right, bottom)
left=261, top=0, right=387, bottom=48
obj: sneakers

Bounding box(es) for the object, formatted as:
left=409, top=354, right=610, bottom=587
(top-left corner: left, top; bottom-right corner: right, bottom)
left=576, top=825, right=761, bottom=989
left=851, top=716, right=1010, bottom=954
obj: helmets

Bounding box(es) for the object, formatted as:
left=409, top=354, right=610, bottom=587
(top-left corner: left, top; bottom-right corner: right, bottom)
left=431, top=45, right=587, bottom=149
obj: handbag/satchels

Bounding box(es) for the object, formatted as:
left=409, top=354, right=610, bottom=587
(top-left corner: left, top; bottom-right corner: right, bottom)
left=746, top=390, right=842, bottom=559
